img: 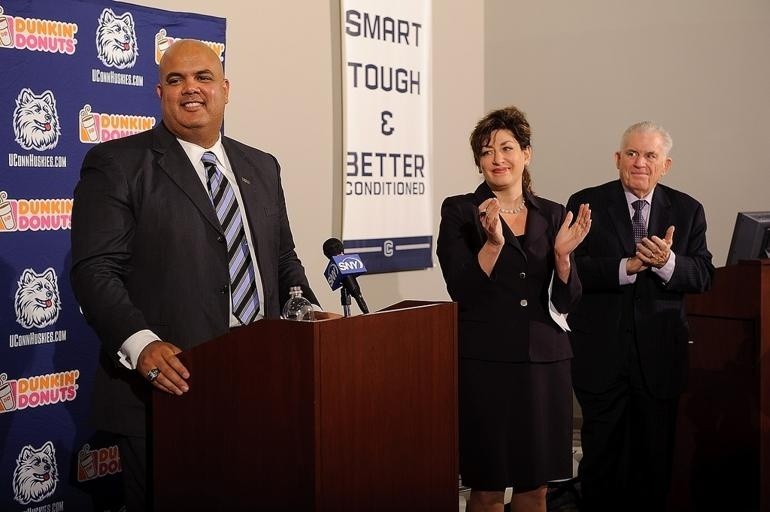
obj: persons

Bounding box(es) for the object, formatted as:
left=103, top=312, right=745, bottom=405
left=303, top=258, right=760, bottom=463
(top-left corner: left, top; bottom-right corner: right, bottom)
left=71, top=35, right=344, bottom=510
left=565, top=122, right=715, bottom=511
left=436, top=104, right=592, bottom=512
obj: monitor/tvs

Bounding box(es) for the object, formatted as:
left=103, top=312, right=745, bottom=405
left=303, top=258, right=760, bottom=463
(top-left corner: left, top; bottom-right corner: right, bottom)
left=726, top=211, right=770, bottom=265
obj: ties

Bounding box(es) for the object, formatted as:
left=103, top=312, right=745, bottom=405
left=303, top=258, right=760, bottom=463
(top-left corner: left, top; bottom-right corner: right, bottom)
left=631, top=200, right=648, bottom=252
left=200, top=152, right=260, bottom=325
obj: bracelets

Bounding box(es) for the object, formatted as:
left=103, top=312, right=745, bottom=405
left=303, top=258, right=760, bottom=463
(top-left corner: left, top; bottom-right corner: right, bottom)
left=495, top=197, right=528, bottom=216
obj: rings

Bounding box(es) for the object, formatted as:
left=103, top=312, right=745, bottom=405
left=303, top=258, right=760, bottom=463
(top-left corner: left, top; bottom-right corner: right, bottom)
left=145, top=367, right=162, bottom=379
left=652, top=253, right=655, bottom=258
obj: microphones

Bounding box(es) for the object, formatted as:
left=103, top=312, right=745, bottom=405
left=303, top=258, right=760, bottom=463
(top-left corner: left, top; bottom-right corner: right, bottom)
left=323, top=238, right=368, bottom=313
